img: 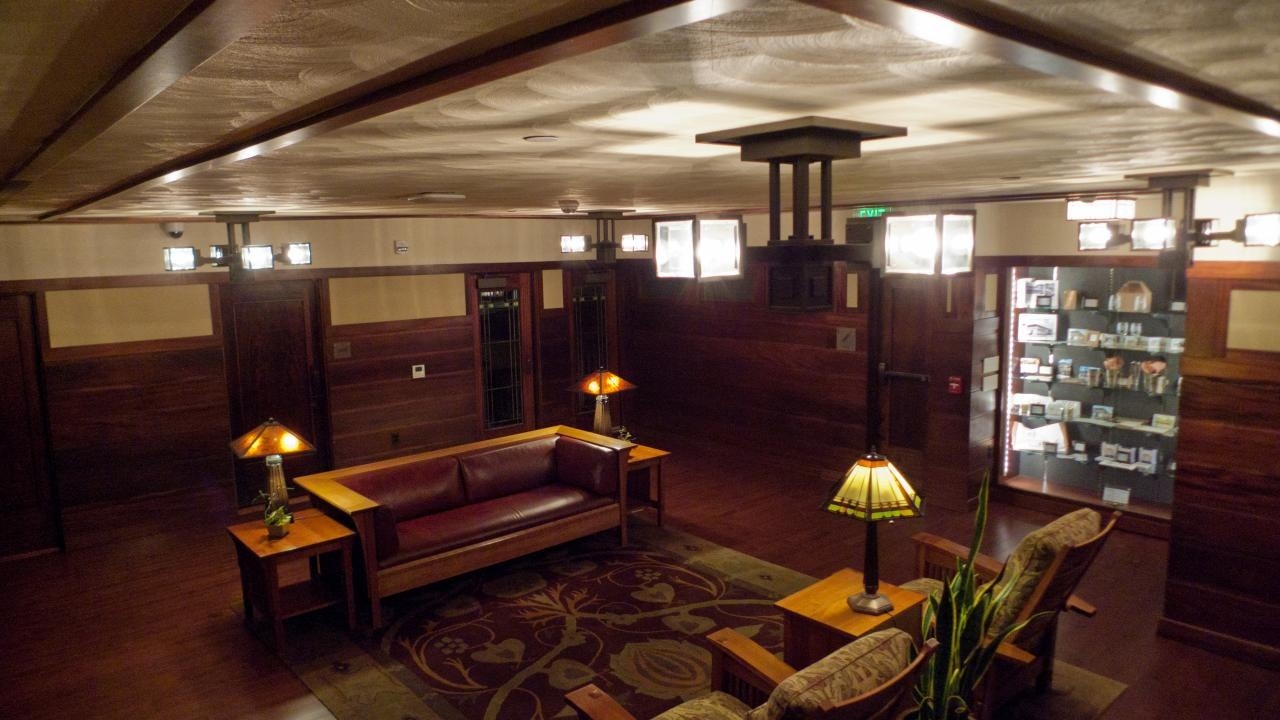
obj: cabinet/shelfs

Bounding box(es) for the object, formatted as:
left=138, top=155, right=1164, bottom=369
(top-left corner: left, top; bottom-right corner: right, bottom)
left=1002, top=255, right=1190, bottom=542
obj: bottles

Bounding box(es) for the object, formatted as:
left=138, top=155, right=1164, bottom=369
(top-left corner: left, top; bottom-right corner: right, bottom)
left=1088, top=368, right=1104, bottom=388
left=1128, top=362, right=1141, bottom=391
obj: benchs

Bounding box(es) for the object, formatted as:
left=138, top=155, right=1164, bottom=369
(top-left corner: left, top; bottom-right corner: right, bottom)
left=289, top=423, right=641, bottom=630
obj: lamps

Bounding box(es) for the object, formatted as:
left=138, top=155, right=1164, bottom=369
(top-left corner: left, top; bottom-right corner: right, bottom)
left=560, top=233, right=593, bottom=253
left=619, top=232, right=649, bottom=254
left=650, top=214, right=748, bottom=285
left=224, top=416, right=324, bottom=523
left=161, top=244, right=199, bottom=273
left=241, top=243, right=276, bottom=272
left=280, top=241, right=315, bottom=268
left=1064, top=192, right=1138, bottom=223
left=208, top=243, right=237, bottom=268
left=563, top=361, right=639, bottom=436
left=816, top=443, right=927, bottom=618
left=1127, top=213, right=1180, bottom=251
left=1193, top=211, right=1280, bottom=250
left=1075, top=218, right=1122, bottom=253
left=874, top=206, right=981, bottom=284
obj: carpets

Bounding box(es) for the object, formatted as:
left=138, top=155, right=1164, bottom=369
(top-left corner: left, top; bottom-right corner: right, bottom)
left=228, top=518, right=1132, bottom=720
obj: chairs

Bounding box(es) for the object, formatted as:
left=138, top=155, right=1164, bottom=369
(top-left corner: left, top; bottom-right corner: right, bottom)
left=896, top=505, right=1125, bottom=720
left=562, top=623, right=947, bottom=720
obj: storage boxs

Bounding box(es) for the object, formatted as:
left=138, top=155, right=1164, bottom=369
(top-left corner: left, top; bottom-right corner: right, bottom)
left=1115, top=279, right=1161, bottom=315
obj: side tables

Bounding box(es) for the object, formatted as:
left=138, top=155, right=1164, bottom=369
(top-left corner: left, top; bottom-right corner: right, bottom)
left=772, top=566, right=932, bottom=707
left=594, top=440, right=674, bottom=534
left=220, top=505, right=363, bottom=657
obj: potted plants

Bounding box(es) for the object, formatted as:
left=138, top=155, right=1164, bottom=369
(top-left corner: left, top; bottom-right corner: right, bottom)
left=896, top=465, right=1057, bottom=720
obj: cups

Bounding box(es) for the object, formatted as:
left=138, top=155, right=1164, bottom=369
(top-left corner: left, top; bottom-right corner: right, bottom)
left=1143, top=373, right=1153, bottom=391
left=1106, top=370, right=1119, bottom=388
left=1130, top=323, right=1142, bottom=336
left=1117, top=322, right=1129, bottom=335
left=1064, top=289, right=1077, bottom=310
